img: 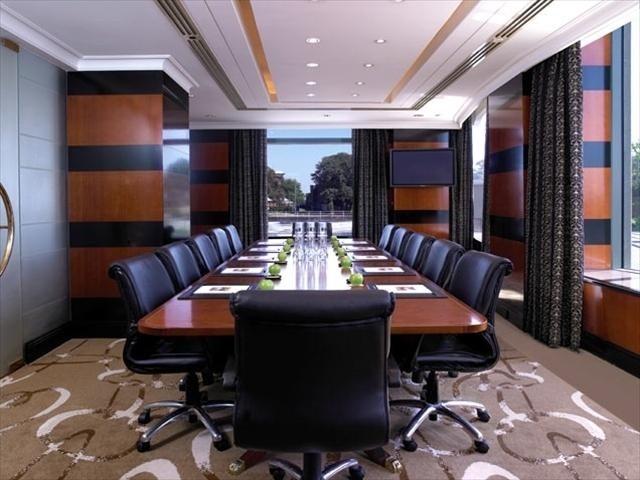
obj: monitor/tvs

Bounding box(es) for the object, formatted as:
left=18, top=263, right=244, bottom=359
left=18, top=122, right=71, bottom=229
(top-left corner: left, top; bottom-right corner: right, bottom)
left=388, top=148, right=458, bottom=188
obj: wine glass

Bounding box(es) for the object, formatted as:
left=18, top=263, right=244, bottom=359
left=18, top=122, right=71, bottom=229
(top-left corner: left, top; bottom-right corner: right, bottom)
left=294, top=264, right=327, bottom=289
left=291, top=230, right=330, bottom=267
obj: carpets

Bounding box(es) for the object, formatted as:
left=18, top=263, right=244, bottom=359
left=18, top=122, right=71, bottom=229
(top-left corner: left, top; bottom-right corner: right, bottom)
left=1, top=334, right=640, bottom=480
